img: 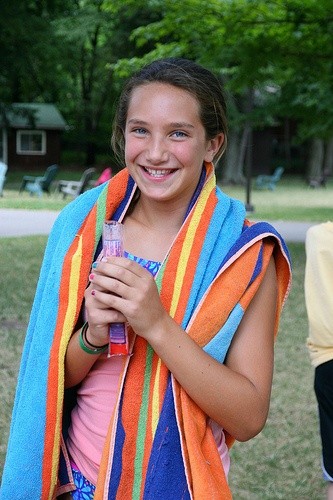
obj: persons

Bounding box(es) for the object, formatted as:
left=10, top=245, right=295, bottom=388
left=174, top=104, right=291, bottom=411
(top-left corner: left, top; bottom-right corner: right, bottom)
left=57, top=58, right=277, bottom=500
left=305, top=219, right=333, bottom=500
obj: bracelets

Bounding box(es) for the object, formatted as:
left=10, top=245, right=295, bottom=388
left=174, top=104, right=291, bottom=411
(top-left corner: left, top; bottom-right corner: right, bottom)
left=78, top=322, right=110, bottom=356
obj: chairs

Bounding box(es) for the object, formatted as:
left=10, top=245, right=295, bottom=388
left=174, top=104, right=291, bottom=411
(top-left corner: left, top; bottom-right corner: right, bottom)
left=19, top=164, right=58, bottom=196
left=257, top=166, right=285, bottom=191
left=308, top=169, right=331, bottom=189
left=57, top=168, right=97, bottom=200
left=88, top=167, right=113, bottom=189
